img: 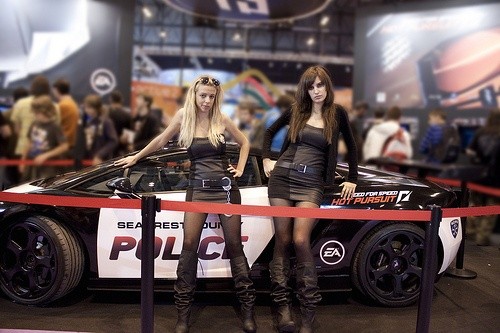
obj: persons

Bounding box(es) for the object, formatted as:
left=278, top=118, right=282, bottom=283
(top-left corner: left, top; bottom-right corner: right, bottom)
left=337, top=101, right=500, bottom=244
left=237, top=95, right=294, bottom=150
left=0, top=76, right=160, bottom=187
left=114, top=75, right=257, bottom=333
left=262, top=67, right=358, bottom=333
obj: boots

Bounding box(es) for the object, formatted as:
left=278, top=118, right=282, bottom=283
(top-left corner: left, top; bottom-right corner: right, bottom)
left=269, top=257, right=297, bottom=333
left=230, top=256, right=257, bottom=333
left=172, top=250, right=198, bottom=333
left=293, top=262, right=322, bottom=333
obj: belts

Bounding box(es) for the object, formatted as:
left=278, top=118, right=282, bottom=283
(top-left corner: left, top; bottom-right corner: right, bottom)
left=188, top=179, right=237, bottom=186
left=273, top=161, right=324, bottom=176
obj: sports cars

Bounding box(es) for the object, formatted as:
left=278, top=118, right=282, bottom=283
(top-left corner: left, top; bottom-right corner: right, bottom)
left=0, top=138, right=463, bottom=308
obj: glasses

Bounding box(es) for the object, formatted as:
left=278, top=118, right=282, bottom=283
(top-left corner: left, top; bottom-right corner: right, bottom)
left=196, top=77, right=220, bottom=87
